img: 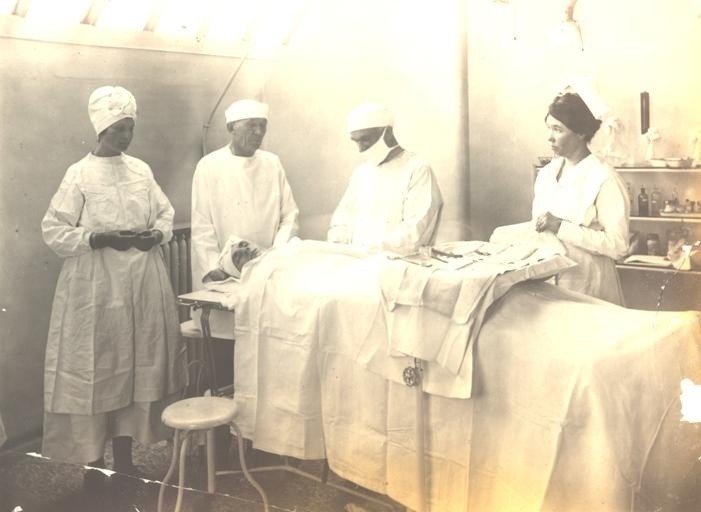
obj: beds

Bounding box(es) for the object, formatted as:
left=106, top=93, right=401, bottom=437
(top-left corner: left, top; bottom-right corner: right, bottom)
left=174, top=287, right=701, bottom=512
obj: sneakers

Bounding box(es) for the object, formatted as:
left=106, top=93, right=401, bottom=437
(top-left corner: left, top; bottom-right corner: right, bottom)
left=85, top=466, right=152, bottom=504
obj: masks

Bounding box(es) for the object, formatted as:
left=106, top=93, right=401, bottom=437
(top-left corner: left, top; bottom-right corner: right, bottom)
left=360, top=129, right=400, bottom=168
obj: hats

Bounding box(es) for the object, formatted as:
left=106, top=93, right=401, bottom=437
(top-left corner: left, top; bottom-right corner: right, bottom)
left=218, top=235, right=242, bottom=278
left=88, top=86, right=136, bottom=136
left=545, top=94, right=601, bottom=143
left=226, top=100, right=268, bottom=125
left=347, top=105, right=393, bottom=132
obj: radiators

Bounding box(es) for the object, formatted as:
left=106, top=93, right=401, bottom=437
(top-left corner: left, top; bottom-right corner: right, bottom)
left=161, top=226, right=200, bottom=386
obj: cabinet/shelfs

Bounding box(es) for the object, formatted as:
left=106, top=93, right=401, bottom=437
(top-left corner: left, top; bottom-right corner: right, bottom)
left=534, top=165, right=701, bottom=276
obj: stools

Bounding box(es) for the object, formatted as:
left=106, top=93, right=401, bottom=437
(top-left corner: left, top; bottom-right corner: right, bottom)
left=157, top=396, right=269, bottom=511
left=179, top=319, right=207, bottom=399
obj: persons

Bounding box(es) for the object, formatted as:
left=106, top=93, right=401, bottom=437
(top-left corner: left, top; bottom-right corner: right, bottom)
left=190, top=98, right=300, bottom=468
left=41, top=86, right=175, bottom=486
left=219, top=232, right=266, bottom=280
left=531, top=86, right=631, bottom=309
left=326, top=101, right=444, bottom=258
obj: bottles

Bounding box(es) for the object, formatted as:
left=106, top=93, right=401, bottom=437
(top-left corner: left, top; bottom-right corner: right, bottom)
left=645, top=233, right=660, bottom=256
left=637, top=184, right=648, bottom=217
left=627, top=182, right=634, bottom=218
left=651, top=184, right=661, bottom=217
